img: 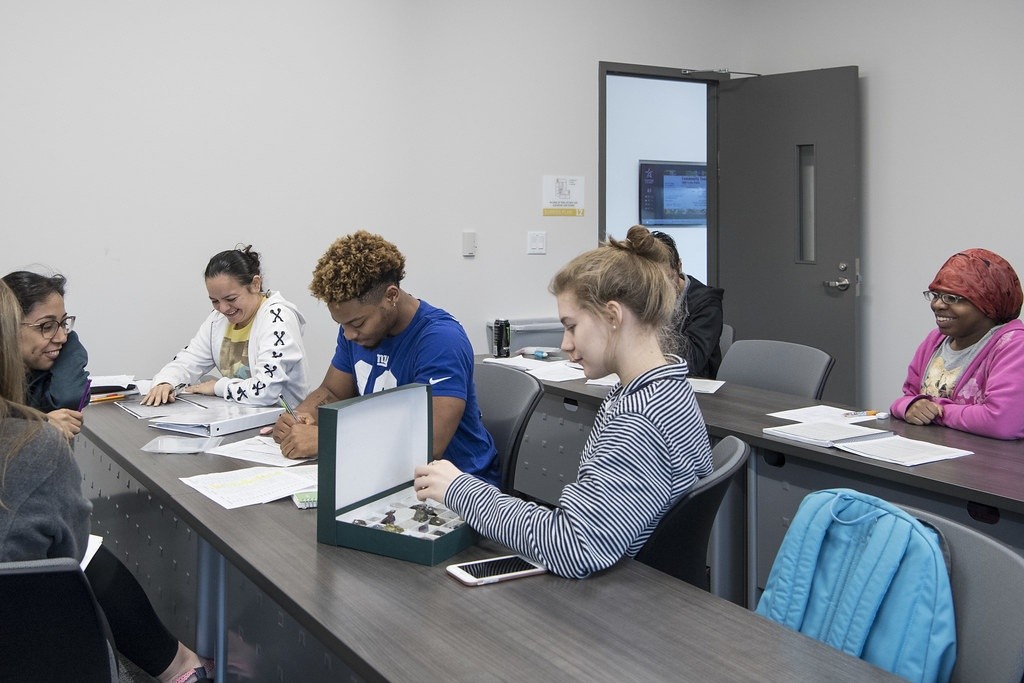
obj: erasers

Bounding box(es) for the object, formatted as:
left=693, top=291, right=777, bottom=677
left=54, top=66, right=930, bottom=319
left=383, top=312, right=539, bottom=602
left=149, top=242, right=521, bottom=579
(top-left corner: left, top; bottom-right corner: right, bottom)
left=260, top=427, right=272, bottom=435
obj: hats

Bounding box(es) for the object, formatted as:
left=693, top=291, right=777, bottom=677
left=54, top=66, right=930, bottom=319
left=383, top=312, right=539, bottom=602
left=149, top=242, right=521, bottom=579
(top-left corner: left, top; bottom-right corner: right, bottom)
left=929, top=248, right=1023, bottom=320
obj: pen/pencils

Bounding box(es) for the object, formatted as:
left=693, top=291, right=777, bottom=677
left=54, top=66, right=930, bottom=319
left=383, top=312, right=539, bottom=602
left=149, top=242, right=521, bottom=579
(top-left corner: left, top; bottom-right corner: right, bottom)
left=170, top=383, right=187, bottom=395
left=90, top=394, right=126, bottom=403
left=278, top=393, right=299, bottom=423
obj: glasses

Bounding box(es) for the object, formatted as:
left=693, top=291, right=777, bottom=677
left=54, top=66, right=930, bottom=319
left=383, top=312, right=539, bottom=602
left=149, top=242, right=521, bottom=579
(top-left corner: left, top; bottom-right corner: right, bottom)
left=923, top=289, right=965, bottom=304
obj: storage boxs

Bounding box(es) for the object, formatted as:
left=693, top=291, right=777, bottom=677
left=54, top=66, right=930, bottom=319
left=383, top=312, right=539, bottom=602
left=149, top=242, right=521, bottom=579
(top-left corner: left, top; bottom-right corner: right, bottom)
left=318, top=382, right=486, bottom=567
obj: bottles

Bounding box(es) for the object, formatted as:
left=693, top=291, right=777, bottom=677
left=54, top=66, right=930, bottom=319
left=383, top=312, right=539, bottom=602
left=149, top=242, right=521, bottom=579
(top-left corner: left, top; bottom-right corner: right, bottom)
left=534, top=350, right=548, bottom=358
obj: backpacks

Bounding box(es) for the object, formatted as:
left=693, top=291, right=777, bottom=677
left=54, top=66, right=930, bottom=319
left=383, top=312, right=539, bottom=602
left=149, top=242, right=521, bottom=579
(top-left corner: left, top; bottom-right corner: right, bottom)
left=754, top=486, right=957, bottom=682
left=21, top=316, right=76, bottom=339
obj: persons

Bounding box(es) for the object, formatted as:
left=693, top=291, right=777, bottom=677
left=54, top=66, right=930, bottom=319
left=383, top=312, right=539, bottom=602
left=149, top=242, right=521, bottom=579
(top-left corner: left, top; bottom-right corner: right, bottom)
left=890, top=248, right=1024, bottom=439
left=273, top=230, right=500, bottom=491
left=650, top=231, right=725, bottom=381
left=0, top=281, right=214, bottom=683
left=414, top=225, right=713, bottom=579
left=140, top=245, right=308, bottom=410
left=0, top=271, right=90, bottom=438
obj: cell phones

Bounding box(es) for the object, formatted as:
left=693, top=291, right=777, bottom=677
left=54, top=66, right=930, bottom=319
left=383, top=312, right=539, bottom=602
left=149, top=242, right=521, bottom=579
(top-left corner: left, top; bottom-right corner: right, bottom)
left=445, top=554, right=547, bottom=586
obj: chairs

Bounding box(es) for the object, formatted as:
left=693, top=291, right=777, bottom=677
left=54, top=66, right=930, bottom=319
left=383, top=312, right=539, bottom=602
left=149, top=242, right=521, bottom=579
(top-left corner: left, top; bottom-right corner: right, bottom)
left=634, top=435, right=751, bottom=596
left=719, top=324, right=835, bottom=401
left=473, top=361, right=545, bottom=496
left=889, top=501, right=1024, bottom=683
left=0, top=557, right=120, bottom=683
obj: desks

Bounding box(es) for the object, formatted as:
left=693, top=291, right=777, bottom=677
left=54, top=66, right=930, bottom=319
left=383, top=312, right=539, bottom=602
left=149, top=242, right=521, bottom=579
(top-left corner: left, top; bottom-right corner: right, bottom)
left=473, top=354, right=1024, bottom=612
left=79, top=393, right=911, bottom=683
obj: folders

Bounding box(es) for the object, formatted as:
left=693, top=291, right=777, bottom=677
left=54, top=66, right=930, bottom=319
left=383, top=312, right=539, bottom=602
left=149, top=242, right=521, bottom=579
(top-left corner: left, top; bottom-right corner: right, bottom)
left=148, top=407, right=286, bottom=438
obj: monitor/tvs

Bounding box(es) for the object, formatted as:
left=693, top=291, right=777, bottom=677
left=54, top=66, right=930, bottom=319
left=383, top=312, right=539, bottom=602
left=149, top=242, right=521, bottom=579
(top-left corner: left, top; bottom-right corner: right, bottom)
left=639, top=159, right=706, bottom=229
